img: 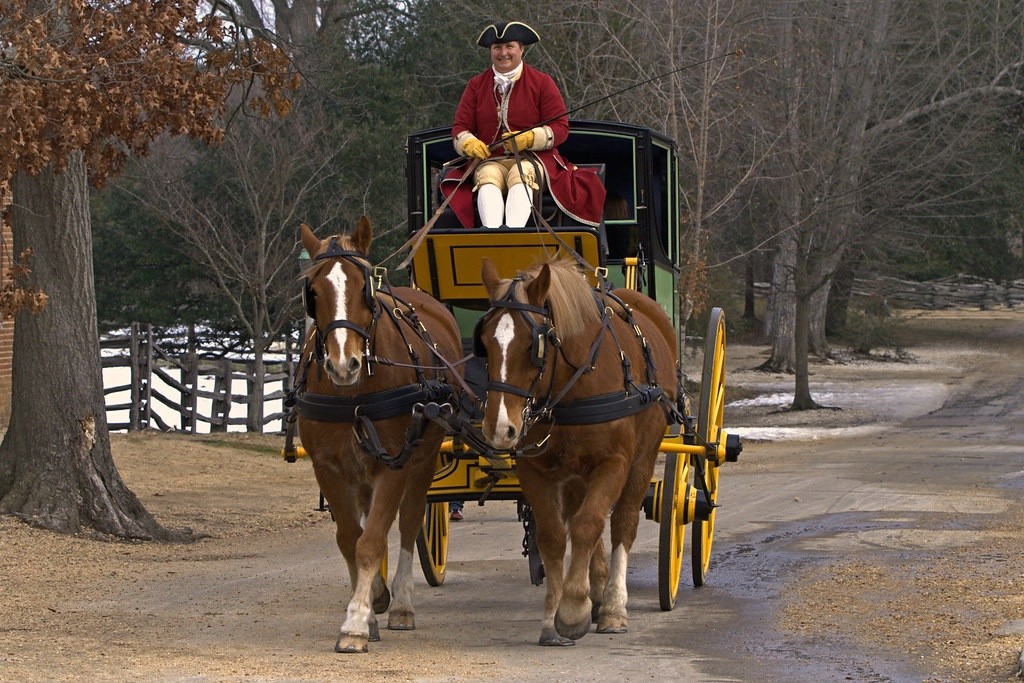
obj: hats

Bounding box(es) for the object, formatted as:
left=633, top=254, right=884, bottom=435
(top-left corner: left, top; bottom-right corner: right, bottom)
left=475, top=20, right=542, bottom=48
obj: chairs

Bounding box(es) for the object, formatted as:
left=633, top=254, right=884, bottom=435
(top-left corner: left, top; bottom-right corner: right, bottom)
left=431, top=164, right=610, bottom=254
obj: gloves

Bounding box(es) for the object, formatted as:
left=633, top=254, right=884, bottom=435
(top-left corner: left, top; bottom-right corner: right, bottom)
left=460, top=137, right=492, bottom=161
left=501, top=129, right=535, bottom=154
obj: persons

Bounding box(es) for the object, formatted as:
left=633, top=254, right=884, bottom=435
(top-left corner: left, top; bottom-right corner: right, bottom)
left=450, top=21, right=569, bottom=229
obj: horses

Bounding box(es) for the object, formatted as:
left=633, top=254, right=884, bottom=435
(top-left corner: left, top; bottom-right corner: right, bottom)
left=292, top=215, right=465, bottom=657
left=470, top=254, right=679, bottom=648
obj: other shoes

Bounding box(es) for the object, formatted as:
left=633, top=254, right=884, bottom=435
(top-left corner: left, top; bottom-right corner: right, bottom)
left=499, top=224, right=510, bottom=229
left=479, top=226, right=488, bottom=229
